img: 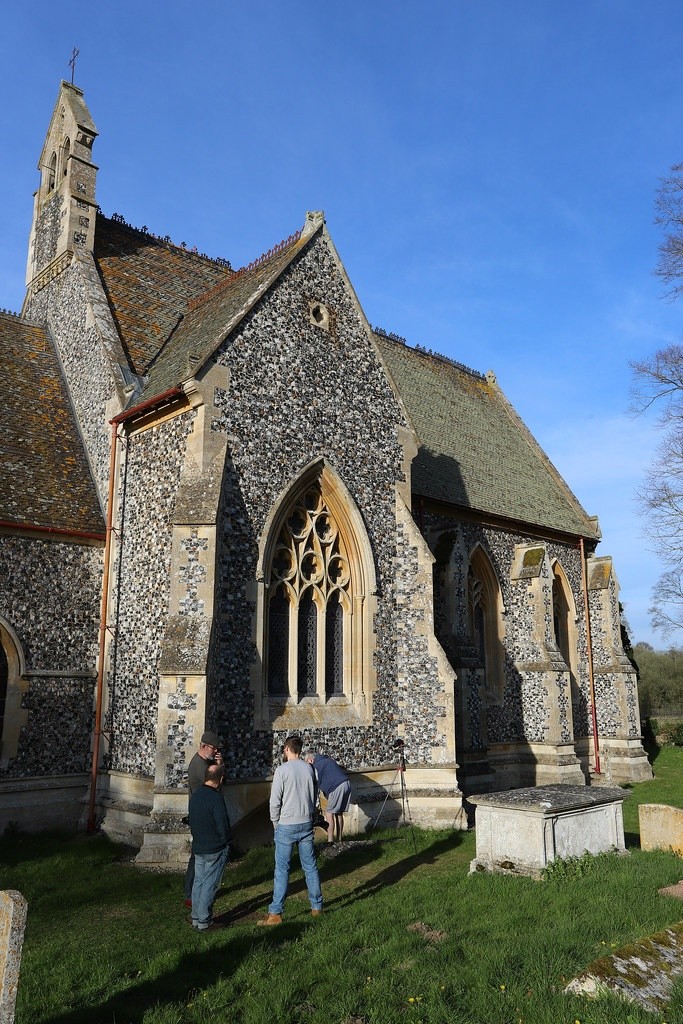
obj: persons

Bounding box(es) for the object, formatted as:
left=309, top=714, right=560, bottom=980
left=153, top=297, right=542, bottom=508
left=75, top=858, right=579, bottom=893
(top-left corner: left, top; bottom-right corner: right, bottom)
left=188, top=765, right=232, bottom=928
left=257, top=737, right=323, bottom=925
left=184, top=728, right=227, bottom=907
left=305, top=753, right=352, bottom=847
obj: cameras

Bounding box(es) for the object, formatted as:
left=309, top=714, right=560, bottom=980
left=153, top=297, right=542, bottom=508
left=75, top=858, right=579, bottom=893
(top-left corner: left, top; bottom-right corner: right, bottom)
left=393, top=739, right=404, bottom=748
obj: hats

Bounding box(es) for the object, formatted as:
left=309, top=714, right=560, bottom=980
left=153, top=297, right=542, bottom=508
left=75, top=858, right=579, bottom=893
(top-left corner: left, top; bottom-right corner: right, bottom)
left=201, top=731, right=224, bottom=748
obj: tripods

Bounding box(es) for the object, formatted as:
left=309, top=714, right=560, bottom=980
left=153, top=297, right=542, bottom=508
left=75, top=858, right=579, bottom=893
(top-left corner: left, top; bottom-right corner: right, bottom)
left=363, top=745, right=418, bottom=856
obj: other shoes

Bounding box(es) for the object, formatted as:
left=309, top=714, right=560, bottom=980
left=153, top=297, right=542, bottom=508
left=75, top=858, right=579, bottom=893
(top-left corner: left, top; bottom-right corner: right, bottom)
left=312, top=909, right=321, bottom=917
left=257, top=914, right=282, bottom=925
left=203, top=923, right=224, bottom=930
left=183, top=900, right=193, bottom=909
